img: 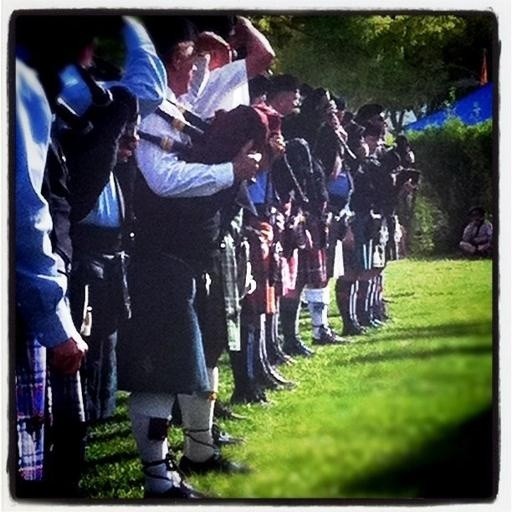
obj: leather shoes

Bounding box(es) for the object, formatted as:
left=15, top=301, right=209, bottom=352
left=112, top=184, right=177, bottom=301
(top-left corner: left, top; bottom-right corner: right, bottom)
left=179, top=446, right=249, bottom=474
left=215, top=401, right=247, bottom=421
left=231, top=386, right=277, bottom=408
left=212, top=423, right=242, bottom=445
left=144, top=480, right=204, bottom=501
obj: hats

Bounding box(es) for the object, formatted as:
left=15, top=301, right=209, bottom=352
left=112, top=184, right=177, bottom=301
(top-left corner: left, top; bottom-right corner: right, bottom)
left=468, top=205, right=482, bottom=213
left=355, top=103, right=383, bottom=123
left=268, top=74, right=298, bottom=92
left=300, top=87, right=329, bottom=116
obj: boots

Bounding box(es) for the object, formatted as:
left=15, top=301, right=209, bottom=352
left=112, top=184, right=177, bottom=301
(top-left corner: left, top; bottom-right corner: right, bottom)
left=305, top=288, right=347, bottom=344
left=335, top=274, right=394, bottom=335
left=227, top=313, right=296, bottom=402
left=281, top=296, right=314, bottom=355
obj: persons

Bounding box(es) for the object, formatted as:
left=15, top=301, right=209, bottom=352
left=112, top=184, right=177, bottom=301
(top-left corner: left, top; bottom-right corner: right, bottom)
left=458, top=205, right=493, bottom=258
left=10, top=10, right=418, bottom=502
